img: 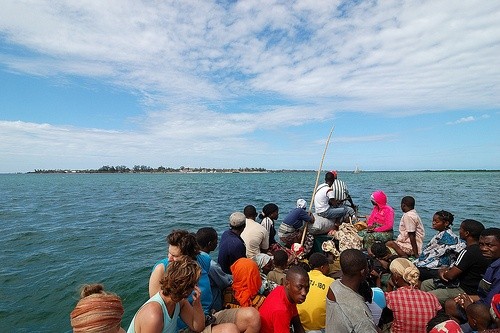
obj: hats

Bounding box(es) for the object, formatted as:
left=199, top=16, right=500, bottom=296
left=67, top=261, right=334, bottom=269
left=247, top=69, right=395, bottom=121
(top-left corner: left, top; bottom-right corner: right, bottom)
left=296, top=199, right=307, bottom=208
left=330, top=171, right=337, bottom=179
left=229, top=212, right=246, bottom=227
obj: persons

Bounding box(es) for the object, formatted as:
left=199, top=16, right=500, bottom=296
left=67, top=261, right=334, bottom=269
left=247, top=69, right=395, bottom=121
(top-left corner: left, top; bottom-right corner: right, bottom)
left=259, top=203, right=299, bottom=268
left=359, top=190, right=394, bottom=255
left=240, top=205, right=273, bottom=274
left=268, top=250, right=287, bottom=285
left=385, top=258, right=443, bottom=333
left=278, top=199, right=315, bottom=249
left=70, top=284, right=126, bottom=333
left=331, top=170, right=353, bottom=206
left=412, top=210, right=500, bottom=333
left=385, top=196, right=425, bottom=258
left=148, top=230, right=262, bottom=333
left=127, top=255, right=205, bottom=333
left=325, top=249, right=377, bottom=333
left=259, top=267, right=310, bottom=333
left=226, top=258, right=266, bottom=309
left=218, top=212, right=247, bottom=275
left=360, top=242, right=395, bottom=324
left=315, top=172, right=347, bottom=227
left=197, top=227, right=234, bottom=310
left=296, top=252, right=335, bottom=333
left=255, top=253, right=280, bottom=298
